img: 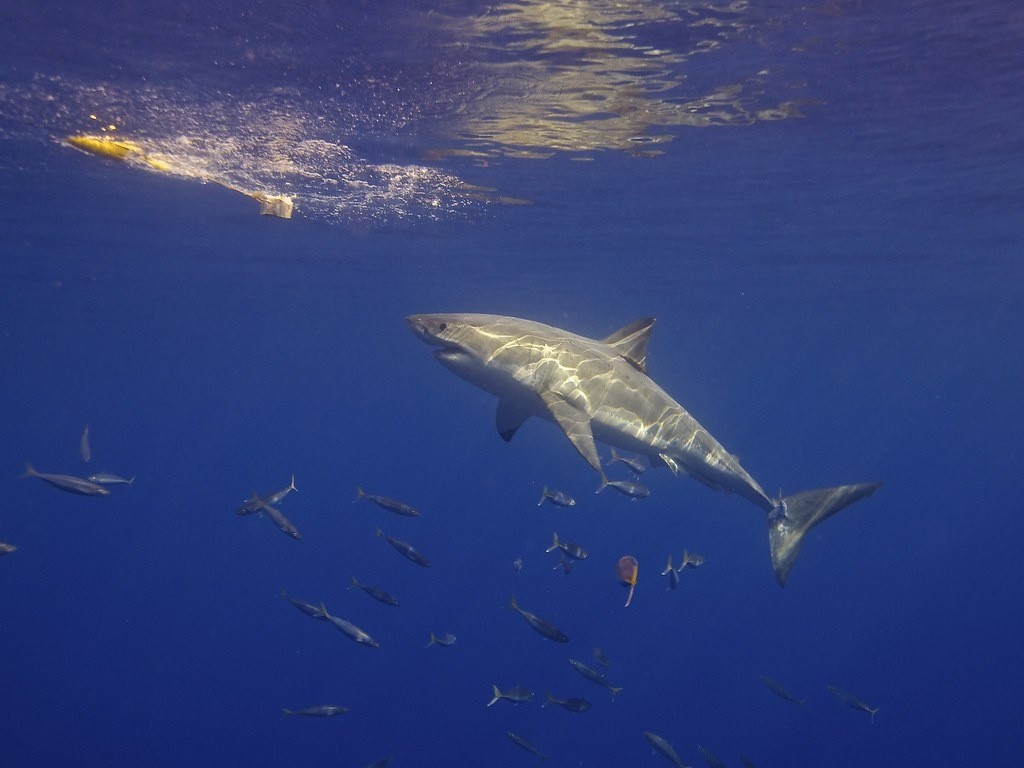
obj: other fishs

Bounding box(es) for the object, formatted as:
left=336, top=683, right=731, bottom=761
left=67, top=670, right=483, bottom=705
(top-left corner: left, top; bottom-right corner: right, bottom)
left=534, top=451, right=705, bottom=606
left=65, top=131, right=166, bottom=178
left=487, top=686, right=537, bottom=707
left=645, top=731, right=684, bottom=768
left=506, top=596, right=571, bottom=643
left=25, top=429, right=430, bottom=647
left=568, top=649, right=624, bottom=695
left=827, top=685, right=882, bottom=725
left=510, top=733, right=551, bottom=759
left=697, top=745, right=723, bottom=768
left=761, top=674, right=808, bottom=708
left=540, top=689, right=593, bottom=714
left=281, top=704, right=348, bottom=719
